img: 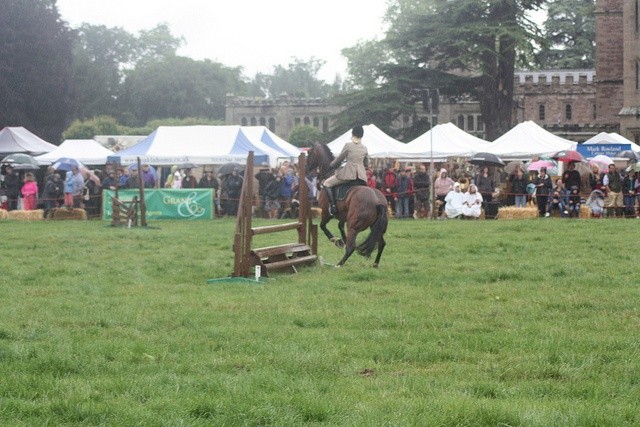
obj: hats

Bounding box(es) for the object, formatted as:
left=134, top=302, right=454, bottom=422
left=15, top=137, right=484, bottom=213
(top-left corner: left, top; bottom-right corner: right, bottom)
left=352, top=125, right=363, bottom=137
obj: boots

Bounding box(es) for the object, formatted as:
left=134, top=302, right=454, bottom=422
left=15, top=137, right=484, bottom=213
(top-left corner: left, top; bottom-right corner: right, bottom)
left=324, top=186, right=338, bottom=215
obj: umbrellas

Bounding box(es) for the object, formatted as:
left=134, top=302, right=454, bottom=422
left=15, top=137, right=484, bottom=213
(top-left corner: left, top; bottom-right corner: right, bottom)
left=128, top=163, right=159, bottom=181
left=551, top=150, right=585, bottom=162
left=217, top=162, right=245, bottom=173
left=503, top=161, right=525, bottom=173
left=0, top=153, right=41, bottom=170
left=177, top=164, right=198, bottom=169
left=468, top=152, right=507, bottom=168
left=615, top=149, right=639, bottom=160
left=51, top=158, right=80, bottom=172
left=527, top=160, right=554, bottom=170
left=589, top=154, right=615, bottom=172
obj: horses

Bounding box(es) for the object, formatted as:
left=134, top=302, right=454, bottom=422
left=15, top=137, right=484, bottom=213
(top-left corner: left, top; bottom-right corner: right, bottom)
left=305, top=139, right=389, bottom=268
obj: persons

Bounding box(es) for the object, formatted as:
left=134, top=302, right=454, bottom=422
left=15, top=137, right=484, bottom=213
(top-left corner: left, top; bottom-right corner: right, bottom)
left=182, top=168, right=196, bottom=188
left=562, top=161, right=581, bottom=200
left=603, top=164, right=623, bottom=209
left=365, top=164, right=415, bottom=217
left=279, top=160, right=317, bottom=219
left=21, top=173, right=38, bottom=211
left=87, top=160, right=131, bottom=209
left=545, top=177, right=568, bottom=218
left=462, top=183, right=483, bottom=217
left=42, top=174, right=58, bottom=218
left=586, top=189, right=604, bottom=217
left=199, top=172, right=224, bottom=217
left=414, top=164, right=431, bottom=218
left=172, top=171, right=182, bottom=188
left=435, top=168, right=455, bottom=220
left=512, top=170, right=528, bottom=209
left=255, top=162, right=275, bottom=217
left=166, top=165, right=177, bottom=187
left=63, top=170, right=73, bottom=206
left=264, top=175, right=283, bottom=218
left=588, top=164, right=610, bottom=196
left=622, top=170, right=636, bottom=217
left=126, top=167, right=142, bottom=188
left=535, top=168, right=550, bottom=217
left=444, top=181, right=464, bottom=219
left=226, top=170, right=243, bottom=212
left=67, top=166, right=85, bottom=206
left=320, top=124, right=371, bottom=217
left=478, top=165, right=495, bottom=208
left=634, top=170, right=640, bottom=219
left=3, top=165, right=20, bottom=210
left=569, top=186, right=581, bottom=218
